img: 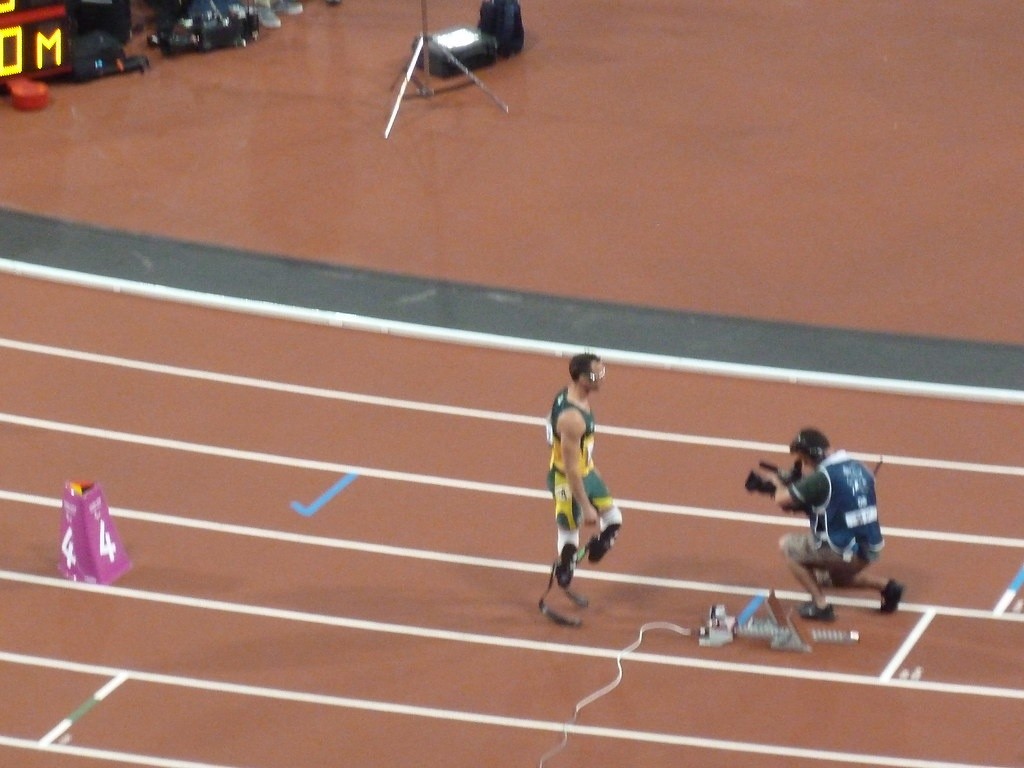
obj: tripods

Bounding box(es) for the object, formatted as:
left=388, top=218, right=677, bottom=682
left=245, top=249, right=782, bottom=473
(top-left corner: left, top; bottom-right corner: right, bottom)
left=382, top=0, right=508, bottom=138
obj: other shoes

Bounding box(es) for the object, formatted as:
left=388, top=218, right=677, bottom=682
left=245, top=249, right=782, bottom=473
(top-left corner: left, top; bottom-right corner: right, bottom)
left=796, top=600, right=837, bottom=622
left=878, top=576, right=904, bottom=613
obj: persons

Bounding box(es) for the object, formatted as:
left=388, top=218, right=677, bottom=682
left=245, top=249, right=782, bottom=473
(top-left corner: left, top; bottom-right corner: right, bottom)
left=536, top=355, right=622, bottom=628
left=751, top=428, right=905, bottom=624
left=61, top=0, right=306, bottom=78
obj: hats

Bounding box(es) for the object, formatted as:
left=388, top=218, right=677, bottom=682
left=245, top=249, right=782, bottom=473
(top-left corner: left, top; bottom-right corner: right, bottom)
left=789, top=430, right=828, bottom=466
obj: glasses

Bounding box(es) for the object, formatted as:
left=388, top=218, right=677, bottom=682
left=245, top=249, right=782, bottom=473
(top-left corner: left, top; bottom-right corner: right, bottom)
left=581, top=366, right=605, bottom=381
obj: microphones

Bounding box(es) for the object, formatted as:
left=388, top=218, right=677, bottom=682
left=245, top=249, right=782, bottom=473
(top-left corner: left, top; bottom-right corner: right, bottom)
left=759, top=459, right=780, bottom=473
